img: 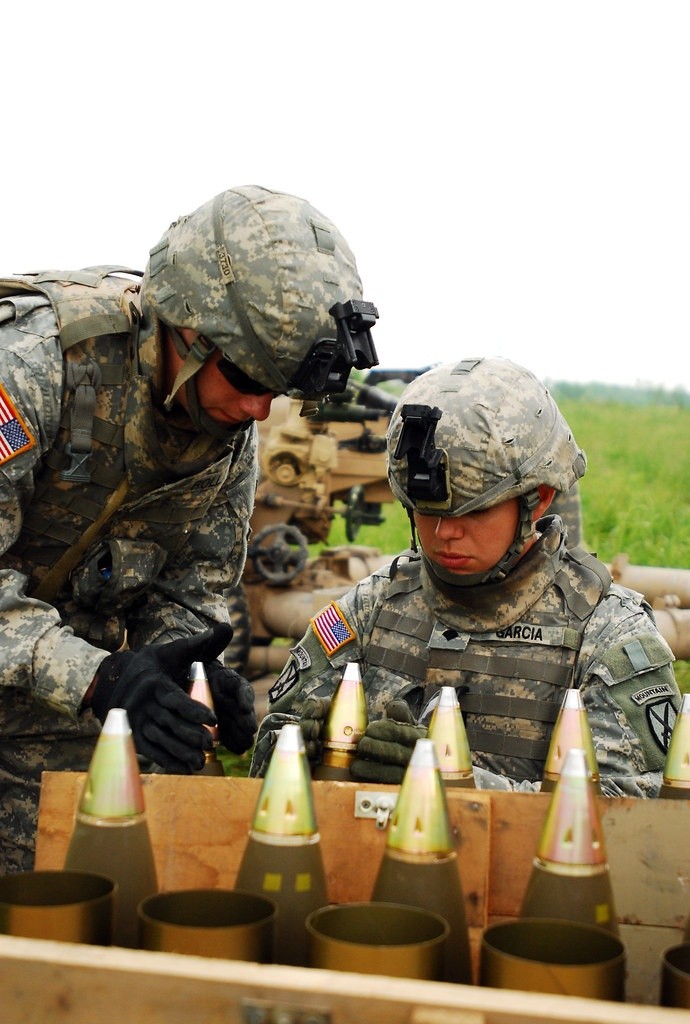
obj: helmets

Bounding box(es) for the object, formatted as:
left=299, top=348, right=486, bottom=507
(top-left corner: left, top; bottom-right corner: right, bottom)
left=385, top=357, right=586, bottom=512
left=143, top=186, right=364, bottom=393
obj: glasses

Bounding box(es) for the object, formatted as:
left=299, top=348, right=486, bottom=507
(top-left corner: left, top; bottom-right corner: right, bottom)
left=216, top=354, right=272, bottom=397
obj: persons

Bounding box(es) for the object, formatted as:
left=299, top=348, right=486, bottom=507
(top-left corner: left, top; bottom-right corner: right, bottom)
left=0, top=184, right=379, bottom=875
left=252, top=358, right=682, bottom=799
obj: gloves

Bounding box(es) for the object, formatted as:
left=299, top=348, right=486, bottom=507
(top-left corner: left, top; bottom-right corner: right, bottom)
left=93, top=622, right=233, bottom=773
left=203, top=656, right=258, bottom=756
left=350, top=699, right=427, bottom=785
left=298, top=693, right=333, bottom=779
